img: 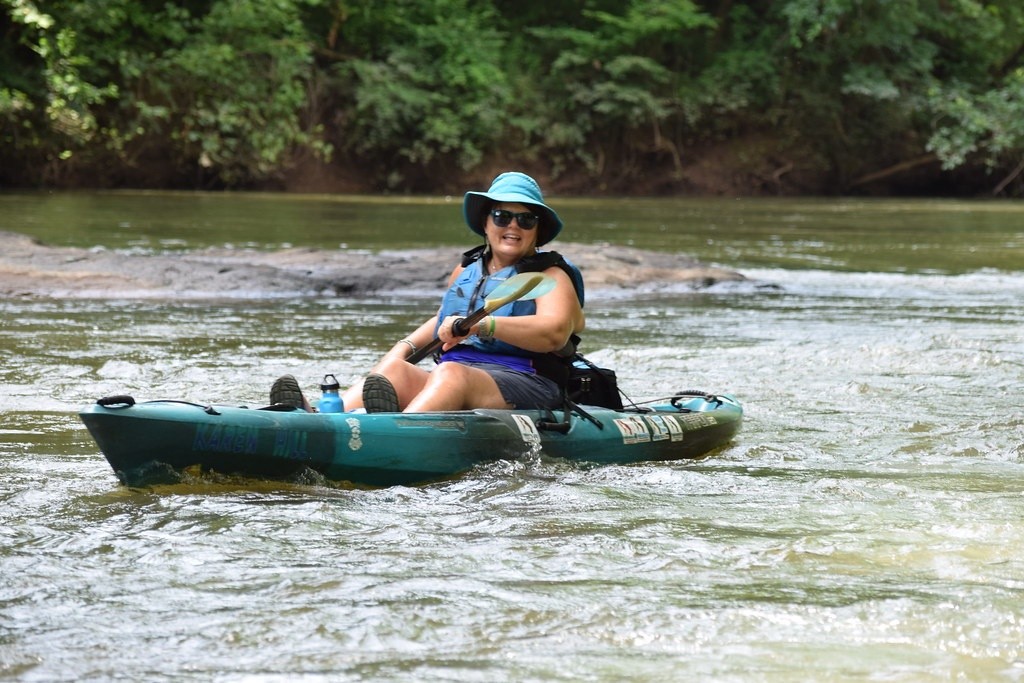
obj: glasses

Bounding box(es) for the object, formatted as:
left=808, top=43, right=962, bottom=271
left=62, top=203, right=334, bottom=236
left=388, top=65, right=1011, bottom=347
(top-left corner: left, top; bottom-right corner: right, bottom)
left=489, top=209, right=541, bottom=230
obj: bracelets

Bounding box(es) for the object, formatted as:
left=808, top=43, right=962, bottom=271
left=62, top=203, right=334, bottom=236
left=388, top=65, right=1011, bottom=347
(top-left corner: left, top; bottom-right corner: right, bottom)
left=487, top=315, right=495, bottom=338
left=475, top=319, right=487, bottom=338
left=398, top=339, right=417, bottom=353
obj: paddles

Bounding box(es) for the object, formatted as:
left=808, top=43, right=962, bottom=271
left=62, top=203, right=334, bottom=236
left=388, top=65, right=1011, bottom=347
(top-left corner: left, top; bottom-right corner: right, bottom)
left=404, top=270, right=558, bottom=364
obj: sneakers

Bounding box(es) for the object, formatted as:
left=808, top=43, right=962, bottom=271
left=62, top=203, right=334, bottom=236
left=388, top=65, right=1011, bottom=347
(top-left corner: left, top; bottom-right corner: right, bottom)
left=269, top=374, right=304, bottom=409
left=362, top=373, right=400, bottom=413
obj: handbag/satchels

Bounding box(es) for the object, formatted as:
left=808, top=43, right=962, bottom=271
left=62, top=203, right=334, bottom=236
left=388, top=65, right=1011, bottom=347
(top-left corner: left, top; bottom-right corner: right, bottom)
left=562, top=348, right=627, bottom=417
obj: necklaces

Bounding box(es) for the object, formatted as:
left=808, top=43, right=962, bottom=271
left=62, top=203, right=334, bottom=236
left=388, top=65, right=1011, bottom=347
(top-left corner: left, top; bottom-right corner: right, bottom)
left=491, top=257, right=517, bottom=269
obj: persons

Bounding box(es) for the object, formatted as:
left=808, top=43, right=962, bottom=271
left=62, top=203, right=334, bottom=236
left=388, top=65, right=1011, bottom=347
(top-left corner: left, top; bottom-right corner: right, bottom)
left=270, top=172, right=584, bottom=414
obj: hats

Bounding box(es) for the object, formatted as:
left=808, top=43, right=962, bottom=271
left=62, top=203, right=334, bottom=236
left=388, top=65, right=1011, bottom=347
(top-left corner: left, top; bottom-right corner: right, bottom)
left=462, top=171, right=564, bottom=248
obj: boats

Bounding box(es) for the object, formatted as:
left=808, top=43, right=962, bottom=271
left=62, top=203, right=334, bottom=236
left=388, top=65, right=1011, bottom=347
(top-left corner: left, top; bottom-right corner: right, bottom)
left=78, top=391, right=744, bottom=487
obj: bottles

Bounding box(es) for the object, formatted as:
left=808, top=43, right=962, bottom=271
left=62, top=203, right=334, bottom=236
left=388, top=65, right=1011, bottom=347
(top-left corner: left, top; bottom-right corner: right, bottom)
left=320, top=374, right=344, bottom=413
